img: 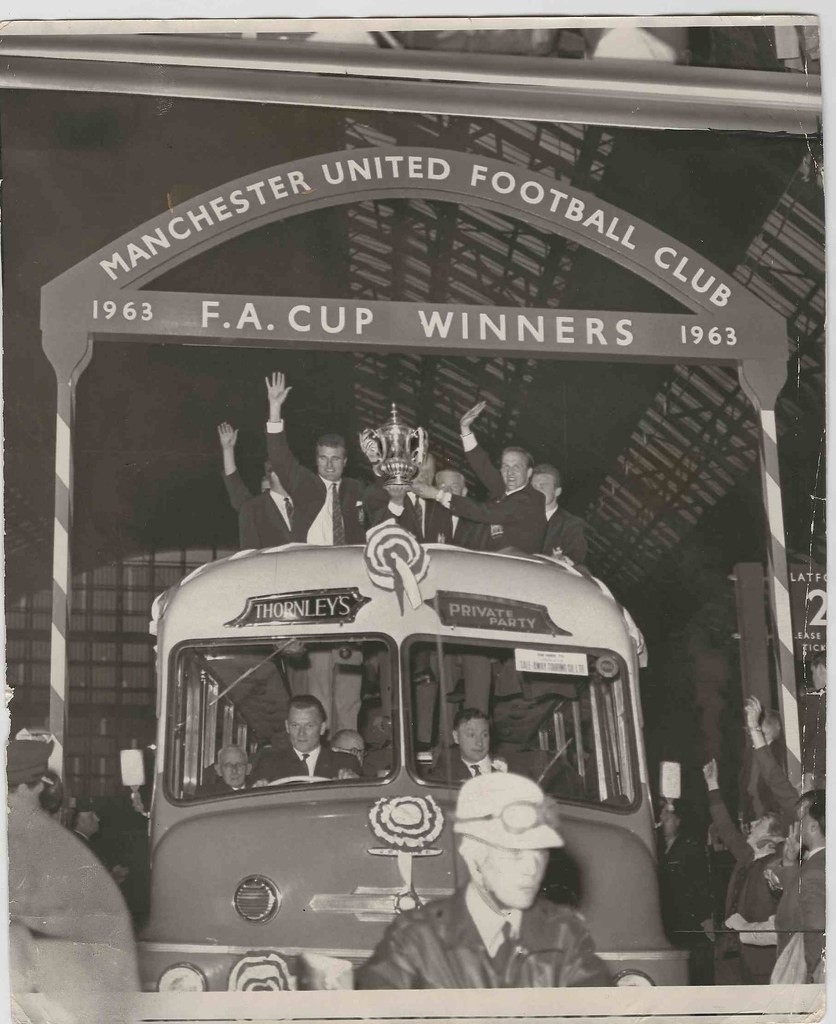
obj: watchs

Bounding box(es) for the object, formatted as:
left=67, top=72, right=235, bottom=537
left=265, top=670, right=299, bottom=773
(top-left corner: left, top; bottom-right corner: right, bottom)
left=749, top=725, right=763, bottom=732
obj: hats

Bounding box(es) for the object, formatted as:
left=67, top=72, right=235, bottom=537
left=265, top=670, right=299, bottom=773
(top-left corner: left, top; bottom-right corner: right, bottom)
left=6, top=740, right=55, bottom=787
left=76, top=796, right=97, bottom=815
left=453, top=771, right=566, bottom=849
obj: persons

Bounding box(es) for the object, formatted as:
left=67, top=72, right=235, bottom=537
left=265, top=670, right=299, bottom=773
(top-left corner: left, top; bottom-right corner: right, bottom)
left=354, top=772, right=655, bottom=989
left=656, top=650, right=825, bottom=986
left=218, top=372, right=590, bottom=751
left=418, top=708, right=536, bottom=786
left=186, top=694, right=368, bottom=800
left=7, top=729, right=149, bottom=994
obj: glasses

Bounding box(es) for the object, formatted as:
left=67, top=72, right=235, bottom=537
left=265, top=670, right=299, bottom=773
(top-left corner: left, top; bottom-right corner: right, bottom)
left=454, top=802, right=565, bottom=834
left=223, top=762, right=248, bottom=770
left=339, top=747, right=368, bottom=757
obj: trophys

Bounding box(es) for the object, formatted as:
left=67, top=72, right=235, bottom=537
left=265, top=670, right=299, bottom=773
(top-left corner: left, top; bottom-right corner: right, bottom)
left=362, top=402, right=428, bottom=485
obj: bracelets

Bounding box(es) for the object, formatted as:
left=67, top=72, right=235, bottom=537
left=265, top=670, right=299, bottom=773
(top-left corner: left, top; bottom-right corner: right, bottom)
left=435, top=488, right=444, bottom=502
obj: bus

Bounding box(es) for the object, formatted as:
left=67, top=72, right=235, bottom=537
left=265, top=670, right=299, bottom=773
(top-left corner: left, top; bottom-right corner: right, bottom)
left=117, top=518, right=694, bottom=992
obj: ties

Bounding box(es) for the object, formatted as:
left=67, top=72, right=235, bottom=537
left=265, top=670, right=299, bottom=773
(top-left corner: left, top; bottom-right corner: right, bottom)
left=494, top=921, right=515, bottom=974
left=471, top=765, right=480, bottom=777
left=285, top=498, right=294, bottom=529
left=415, top=495, right=423, bottom=528
left=331, top=484, right=345, bottom=546
left=302, top=753, right=310, bottom=777
